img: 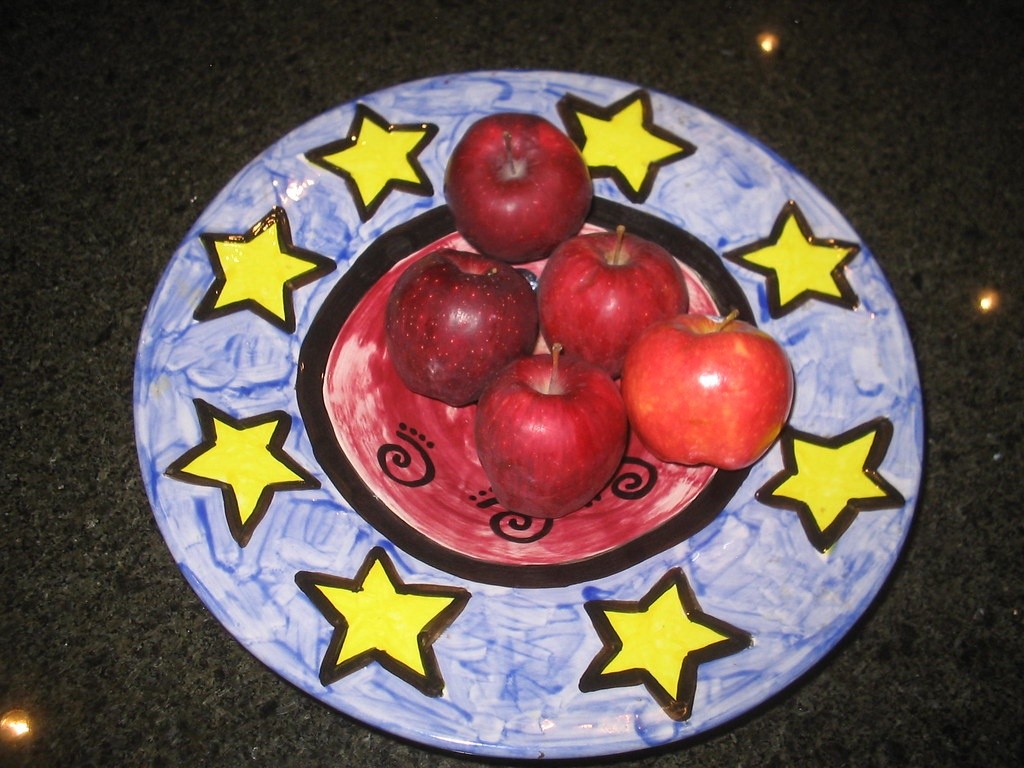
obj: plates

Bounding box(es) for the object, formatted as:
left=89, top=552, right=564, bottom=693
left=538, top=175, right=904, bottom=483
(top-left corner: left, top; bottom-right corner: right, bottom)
left=131, top=69, right=924, bottom=761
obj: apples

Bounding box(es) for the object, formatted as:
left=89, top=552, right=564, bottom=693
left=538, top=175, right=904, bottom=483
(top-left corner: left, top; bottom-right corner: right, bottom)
left=534, top=224, right=689, bottom=380
left=621, top=308, right=794, bottom=470
left=443, top=112, right=593, bottom=263
left=386, top=247, right=538, bottom=407
left=473, top=343, right=627, bottom=519
